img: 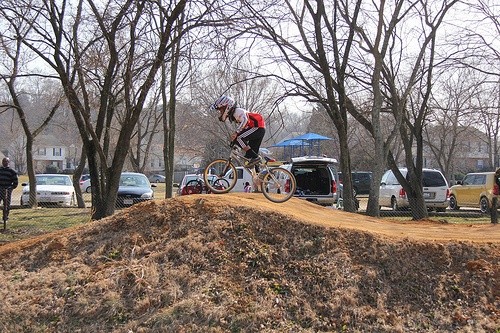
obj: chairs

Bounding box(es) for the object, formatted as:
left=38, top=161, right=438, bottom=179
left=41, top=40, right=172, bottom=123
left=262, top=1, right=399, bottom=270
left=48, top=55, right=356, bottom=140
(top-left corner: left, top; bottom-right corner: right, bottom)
left=37, top=181, right=46, bottom=185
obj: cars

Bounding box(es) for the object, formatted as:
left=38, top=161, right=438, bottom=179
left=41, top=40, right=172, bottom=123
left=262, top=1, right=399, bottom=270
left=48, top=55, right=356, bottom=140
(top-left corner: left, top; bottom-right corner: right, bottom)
left=21, top=173, right=77, bottom=208
left=449, top=168, right=500, bottom=214
left=149, top=174, right=166, bottom=183
left=78, top=174, right=107, bottom=193
left=115, top=172, right=157, bottom=208
left=173, top=174, right=225, bottom=197
left=379, top=167, right=450, bottom=211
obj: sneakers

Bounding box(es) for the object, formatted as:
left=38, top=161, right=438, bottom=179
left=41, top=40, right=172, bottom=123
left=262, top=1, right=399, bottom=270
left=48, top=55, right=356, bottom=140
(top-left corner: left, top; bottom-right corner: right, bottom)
left=246, top=156, right=262, bottom=168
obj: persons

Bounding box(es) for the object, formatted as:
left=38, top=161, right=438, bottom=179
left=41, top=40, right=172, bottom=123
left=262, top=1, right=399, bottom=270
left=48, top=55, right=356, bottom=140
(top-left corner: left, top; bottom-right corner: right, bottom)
left=0, top=158, right=18, bottom=219
left=244, top=182, right=251, bottom=192
left=210, top=96, right=266, bottom=181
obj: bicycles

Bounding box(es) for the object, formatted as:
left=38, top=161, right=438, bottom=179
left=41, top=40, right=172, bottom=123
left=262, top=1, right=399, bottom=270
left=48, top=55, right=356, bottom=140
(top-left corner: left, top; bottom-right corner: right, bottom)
left=203, top=137, right=296, bottom=204
left=186, top=169, right=230, bottom=194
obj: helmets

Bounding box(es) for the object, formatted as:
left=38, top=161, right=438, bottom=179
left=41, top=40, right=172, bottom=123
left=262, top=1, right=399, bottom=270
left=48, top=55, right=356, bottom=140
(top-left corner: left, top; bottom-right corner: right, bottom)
left=215, top=96, right=235, bottom=121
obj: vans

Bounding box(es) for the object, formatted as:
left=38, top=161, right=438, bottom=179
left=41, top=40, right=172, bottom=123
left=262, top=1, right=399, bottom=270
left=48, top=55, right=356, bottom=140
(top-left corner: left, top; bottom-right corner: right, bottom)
left=219, top=156, right=338, bottom=206
left=337, top=172, right=372, bottom=197
